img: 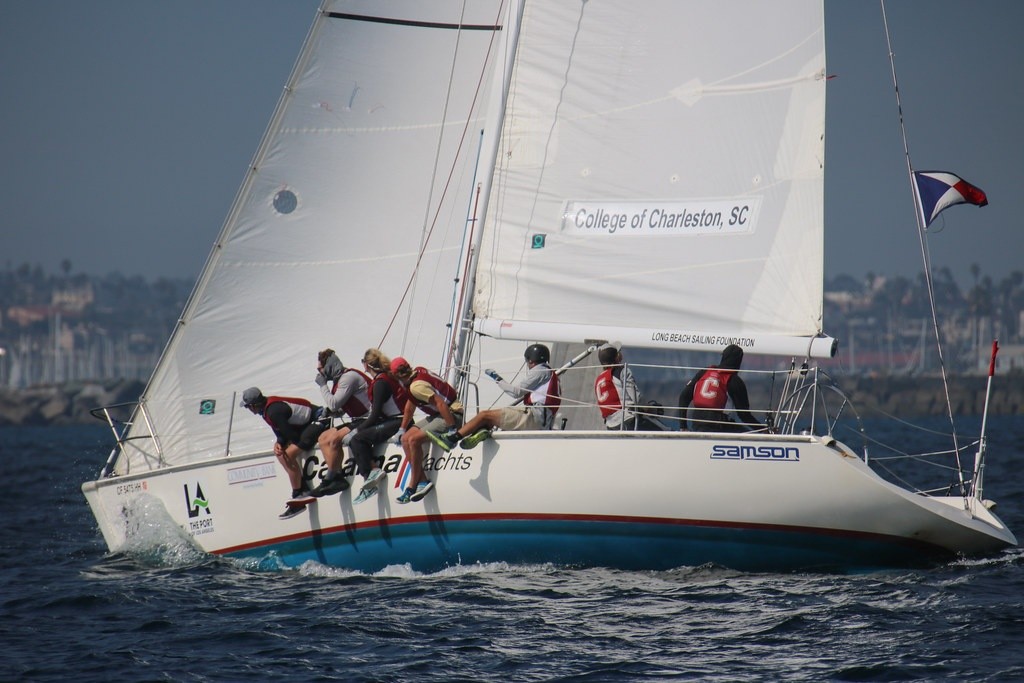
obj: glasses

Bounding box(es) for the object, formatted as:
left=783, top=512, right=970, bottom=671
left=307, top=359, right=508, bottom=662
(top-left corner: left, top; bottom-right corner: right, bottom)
left=317, top=367, right=323, bottom=372
left=361, top=359, right=367, bottom=364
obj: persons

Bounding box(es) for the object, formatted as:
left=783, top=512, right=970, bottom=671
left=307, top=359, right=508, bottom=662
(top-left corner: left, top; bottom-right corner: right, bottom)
left=595, top=341, right=665, bottom=431
left=678, top=344, right=770, bottom=434
left=339, top=348, right=415, bottom=506
left=389, top=357, right=465, bottom=504
left=307, top=348, right=374, bottom=497
left=240, top=387, right=333, bottom=520
left=425, top=344, right=562, bottom=452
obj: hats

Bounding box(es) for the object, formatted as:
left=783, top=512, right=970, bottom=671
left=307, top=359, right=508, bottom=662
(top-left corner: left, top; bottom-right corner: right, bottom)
left=598, top=340, right=622, bottom=363
left=389, top=357, right=408, bottom=375
left=239, top=387, right=262, bottom=407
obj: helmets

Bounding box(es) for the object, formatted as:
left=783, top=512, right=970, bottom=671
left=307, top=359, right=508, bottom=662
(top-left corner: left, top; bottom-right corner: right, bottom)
left=524, top=345, right=549, bottom=363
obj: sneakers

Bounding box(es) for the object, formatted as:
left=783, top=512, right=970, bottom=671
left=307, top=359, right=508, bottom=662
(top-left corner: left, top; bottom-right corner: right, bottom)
left=278, top=505, right=307, bottom=520
left=352, top=467, right=387, bottom=504
left=285, top=489, right=316, bottom=505
left=460, top=427, right=489, bottom=449
left=425, top=428, right=454, bottom=453
left=396, top=480, right=433, bottom=504
left=308, top=478, right=350, bottom=498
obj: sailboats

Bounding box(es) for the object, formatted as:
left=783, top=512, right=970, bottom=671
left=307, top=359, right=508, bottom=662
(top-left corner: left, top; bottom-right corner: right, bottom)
left=81, top=0, right=1018, bottom=576
